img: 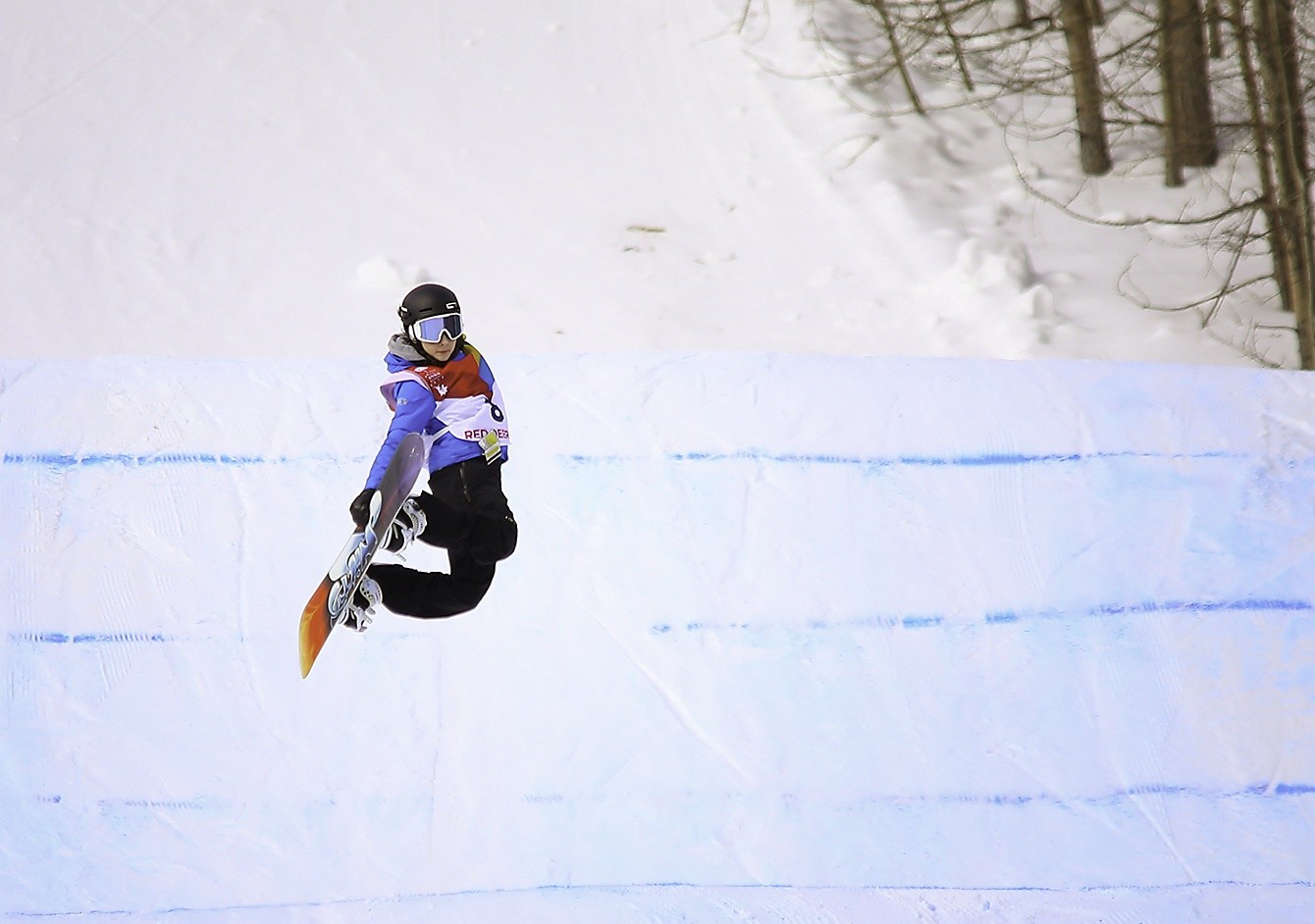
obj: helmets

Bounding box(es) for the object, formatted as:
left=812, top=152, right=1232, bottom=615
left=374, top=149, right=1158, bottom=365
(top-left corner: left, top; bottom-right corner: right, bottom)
left=401, top=284, right=460, bottom=325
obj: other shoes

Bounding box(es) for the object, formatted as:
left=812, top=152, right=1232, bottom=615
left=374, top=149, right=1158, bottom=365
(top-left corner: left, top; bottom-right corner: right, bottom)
left=339, top=574, right=382, bottom=632
left=378, top=497, right=427, bottom=556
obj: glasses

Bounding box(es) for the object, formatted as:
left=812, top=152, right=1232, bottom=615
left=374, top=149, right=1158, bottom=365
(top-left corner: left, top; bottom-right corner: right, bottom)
left=413, top=313, right=463, bottom=343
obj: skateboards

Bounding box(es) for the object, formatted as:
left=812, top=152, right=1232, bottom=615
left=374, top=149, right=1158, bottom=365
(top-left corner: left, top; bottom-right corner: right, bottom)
left=297, top=432, right=427, bottom=681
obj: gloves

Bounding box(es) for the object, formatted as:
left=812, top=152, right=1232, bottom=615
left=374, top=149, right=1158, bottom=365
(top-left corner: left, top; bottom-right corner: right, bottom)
left=351, top=489, right=374, bottom=527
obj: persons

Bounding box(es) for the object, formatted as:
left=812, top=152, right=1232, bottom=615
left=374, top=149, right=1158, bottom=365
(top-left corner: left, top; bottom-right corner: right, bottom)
left=343, top=283, right=518, bottom=629
